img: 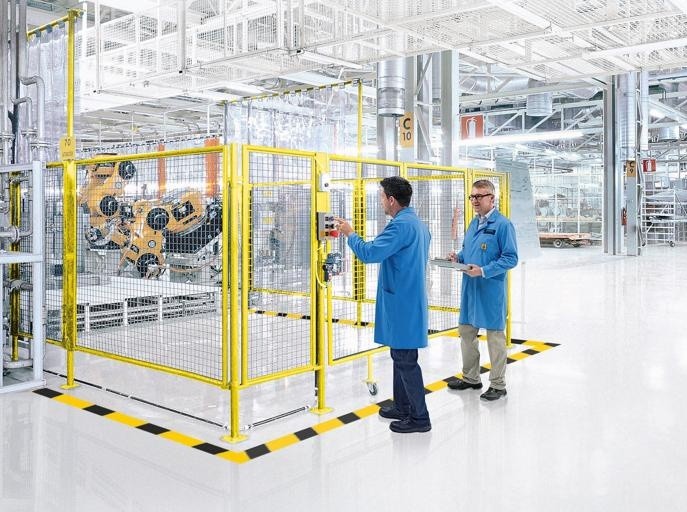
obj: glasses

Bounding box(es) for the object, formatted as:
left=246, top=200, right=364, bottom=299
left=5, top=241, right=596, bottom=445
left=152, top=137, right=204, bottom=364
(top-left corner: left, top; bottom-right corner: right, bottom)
left=468, top=193, right=492, bottom=201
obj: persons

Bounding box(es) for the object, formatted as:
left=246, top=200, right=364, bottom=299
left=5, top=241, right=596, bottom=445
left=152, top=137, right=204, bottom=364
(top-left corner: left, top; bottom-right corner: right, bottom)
left=445, top=179, right=519, bottom=402
left=331, top=176, right=432, bottom=433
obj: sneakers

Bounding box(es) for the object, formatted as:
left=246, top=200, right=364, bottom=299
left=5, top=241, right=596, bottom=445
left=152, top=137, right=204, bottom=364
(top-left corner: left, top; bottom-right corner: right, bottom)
left=390, top=417, right=431, bottom=434
left=447, top=379, right=483, bottom=390
left=379, top=405, right=408, bottom=420
left=480, top=387, right=508, bottom=403
